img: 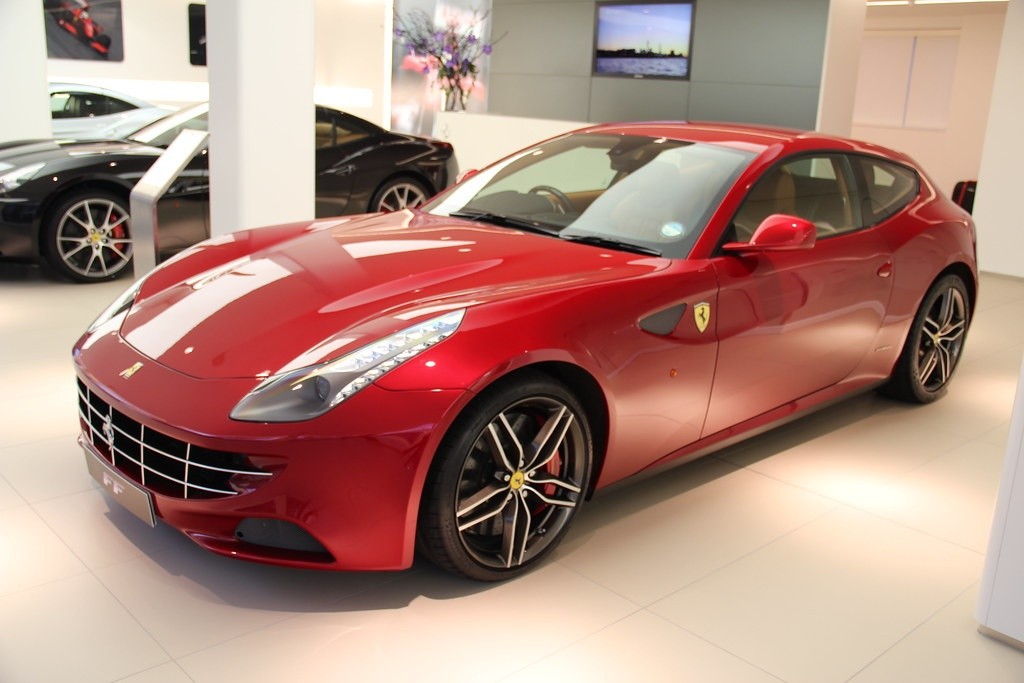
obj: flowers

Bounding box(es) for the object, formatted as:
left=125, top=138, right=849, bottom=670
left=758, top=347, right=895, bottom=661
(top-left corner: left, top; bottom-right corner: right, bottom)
left=393, top=5, right=508, bottom=110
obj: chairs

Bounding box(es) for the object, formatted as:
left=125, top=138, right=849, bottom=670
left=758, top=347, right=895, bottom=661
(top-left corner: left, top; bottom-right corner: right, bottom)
left=613, top=159, right=687, bottom=232
left=730, top=164, right=803, bottom=244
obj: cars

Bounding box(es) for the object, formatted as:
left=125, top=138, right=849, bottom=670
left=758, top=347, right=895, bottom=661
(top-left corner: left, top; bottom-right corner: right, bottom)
left=0, top=98, right=459, bottom=284
left=48, top=80, right=181, bottom=147
left=72, top=120, right=981, bottom=581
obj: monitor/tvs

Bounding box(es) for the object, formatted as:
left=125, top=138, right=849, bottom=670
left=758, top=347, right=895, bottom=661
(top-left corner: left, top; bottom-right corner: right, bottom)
left=591, top=0, right=697, bottom=80
